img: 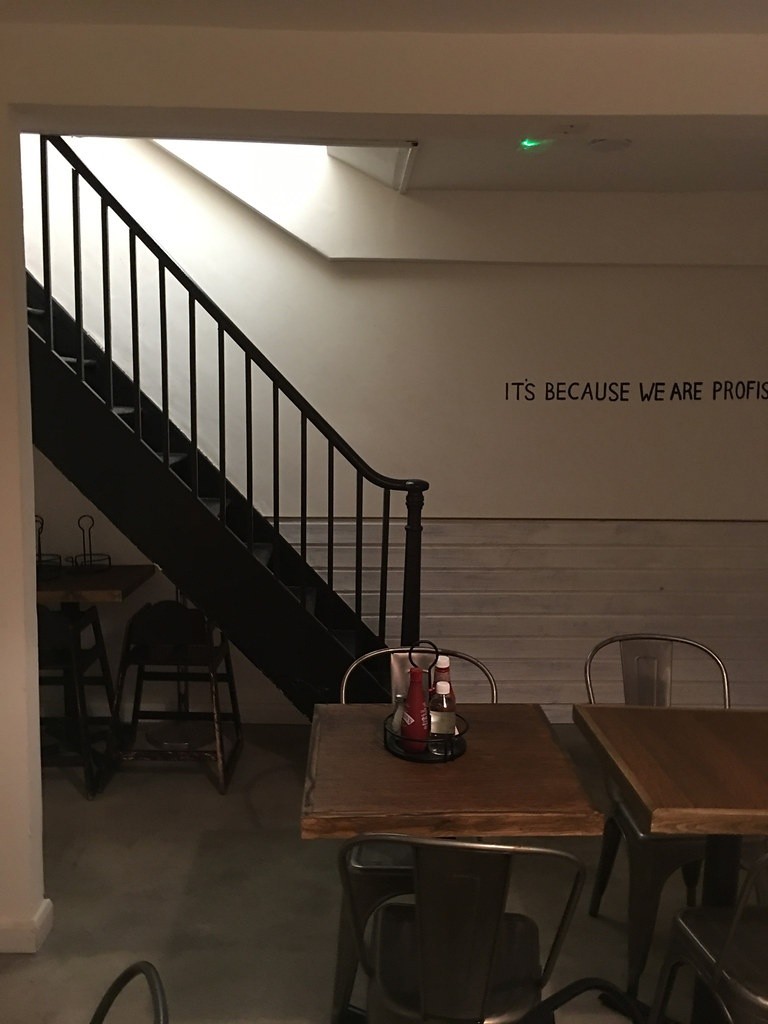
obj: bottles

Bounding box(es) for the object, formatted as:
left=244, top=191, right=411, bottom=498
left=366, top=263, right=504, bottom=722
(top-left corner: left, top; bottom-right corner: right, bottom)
left=401, top=668, right=429, bottom=752
left=392, top=693, right=405, bottom=732
left=428, top=681, right=456, bottom=755
left=433, top=655, right=455, bottom=703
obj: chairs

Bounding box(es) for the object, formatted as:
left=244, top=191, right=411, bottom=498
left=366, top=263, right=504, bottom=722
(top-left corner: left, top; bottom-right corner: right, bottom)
left=36, top=601, right=245, bottom=802
left=329, top=633, right=768, bottom=1024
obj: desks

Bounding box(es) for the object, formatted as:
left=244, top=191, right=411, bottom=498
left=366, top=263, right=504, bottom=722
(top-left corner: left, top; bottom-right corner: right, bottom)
left=299, top=702, right=768, bottom=897
left=36, top=564, right=157, bottom=769
